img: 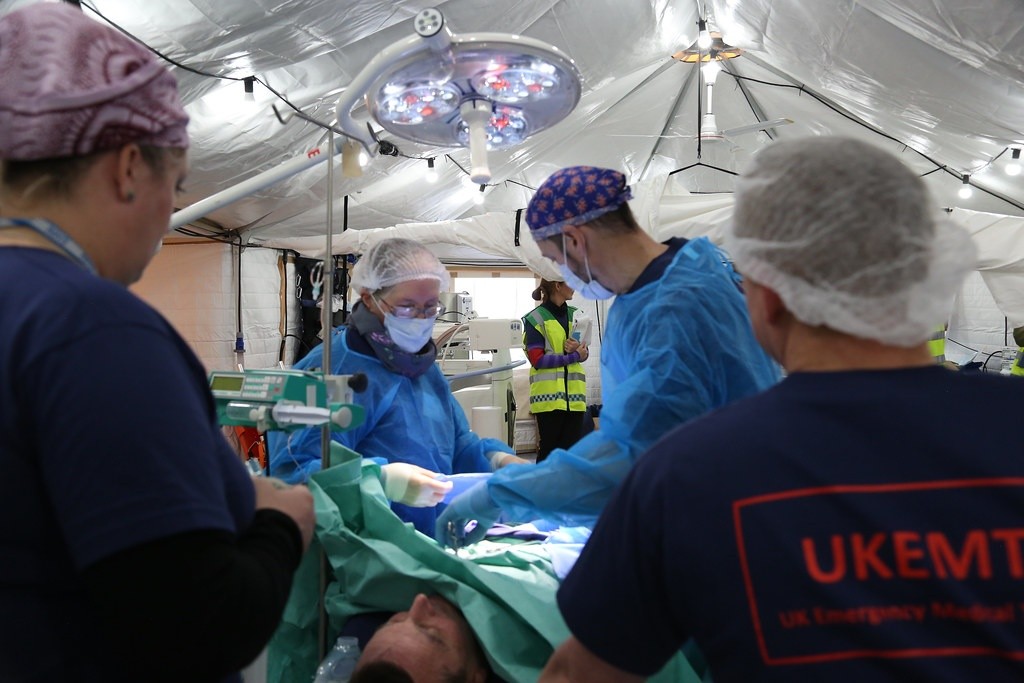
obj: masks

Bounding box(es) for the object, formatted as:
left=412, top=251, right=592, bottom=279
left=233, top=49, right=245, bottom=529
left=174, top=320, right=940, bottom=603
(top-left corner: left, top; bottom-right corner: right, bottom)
left=559, top=231, right=618, bottom=300
left=371, top=294, right=438, bottom=353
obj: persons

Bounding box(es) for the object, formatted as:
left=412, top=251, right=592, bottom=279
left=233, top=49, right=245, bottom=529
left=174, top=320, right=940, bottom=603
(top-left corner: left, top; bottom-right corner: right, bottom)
left=434, top=166, right=785, bottom=550
left=521, top=276, right=593, bottom=464
left=1, top=2, right=315, bottom=683
left=349, top=593, right=493, bottom=683
left=270, top=239, right=533, bottom=541
left=540, top=136, right=1024, bottom=683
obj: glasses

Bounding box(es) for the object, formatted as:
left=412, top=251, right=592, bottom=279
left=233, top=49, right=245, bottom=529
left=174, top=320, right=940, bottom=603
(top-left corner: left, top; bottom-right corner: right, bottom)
left=379, top=297, right=447, bottom=318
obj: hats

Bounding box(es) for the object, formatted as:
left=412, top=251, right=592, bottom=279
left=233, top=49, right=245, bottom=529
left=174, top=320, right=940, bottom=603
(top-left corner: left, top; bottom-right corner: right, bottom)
left=524, top=165, right=635, bottom=241
left=0, top=1, right=190, bottom=160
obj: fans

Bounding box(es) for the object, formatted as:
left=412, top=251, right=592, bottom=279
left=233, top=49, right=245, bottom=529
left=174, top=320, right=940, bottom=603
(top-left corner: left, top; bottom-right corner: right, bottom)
left=609, top=57, right=794, bottom=158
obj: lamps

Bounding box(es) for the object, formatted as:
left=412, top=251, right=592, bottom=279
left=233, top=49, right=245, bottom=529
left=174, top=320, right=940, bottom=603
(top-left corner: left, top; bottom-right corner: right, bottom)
left=363, top=8, right=582, bottom=186
